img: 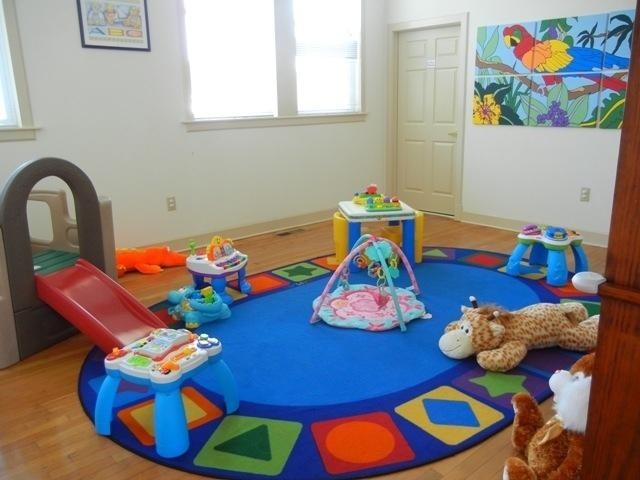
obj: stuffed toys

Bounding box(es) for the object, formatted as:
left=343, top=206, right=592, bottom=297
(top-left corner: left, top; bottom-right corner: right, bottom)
left=438, top=294, right=601, bottom=371
left=116, top=244, right=187, bottom=275
left=502, top=346, right=597, bottom=480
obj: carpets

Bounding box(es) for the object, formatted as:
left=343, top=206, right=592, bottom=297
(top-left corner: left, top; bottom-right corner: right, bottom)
left=78, top=246, right=601, bottom=479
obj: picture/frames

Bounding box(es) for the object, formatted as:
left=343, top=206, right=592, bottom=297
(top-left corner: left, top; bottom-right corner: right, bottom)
left=76, top=0, right=151, bottom=52
